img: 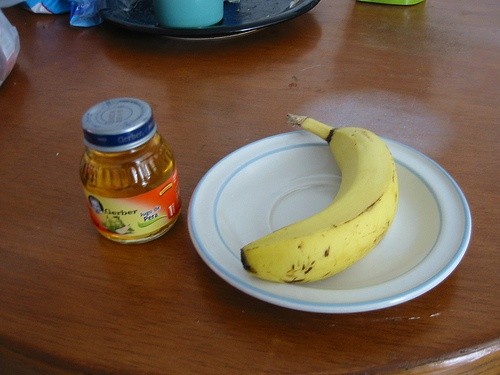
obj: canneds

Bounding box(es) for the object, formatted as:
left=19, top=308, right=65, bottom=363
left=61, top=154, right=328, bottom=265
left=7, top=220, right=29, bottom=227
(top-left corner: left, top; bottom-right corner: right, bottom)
left=79, top=97, right=181, bottom=244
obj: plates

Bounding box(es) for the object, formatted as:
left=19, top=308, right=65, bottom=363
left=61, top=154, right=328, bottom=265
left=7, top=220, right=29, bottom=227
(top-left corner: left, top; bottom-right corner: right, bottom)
left=95, top=0, right=320, bottom=43
left=187, top=129, right=472, bottom=314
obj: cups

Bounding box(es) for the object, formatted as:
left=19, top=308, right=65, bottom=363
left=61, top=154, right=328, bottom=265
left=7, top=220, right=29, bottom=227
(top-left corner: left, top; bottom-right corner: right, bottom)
left=153, top=0, right=224, bottom=29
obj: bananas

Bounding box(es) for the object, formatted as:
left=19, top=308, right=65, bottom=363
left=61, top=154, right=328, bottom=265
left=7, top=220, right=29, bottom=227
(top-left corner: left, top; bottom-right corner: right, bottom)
left=240, top=112, right=397, bottom=284
